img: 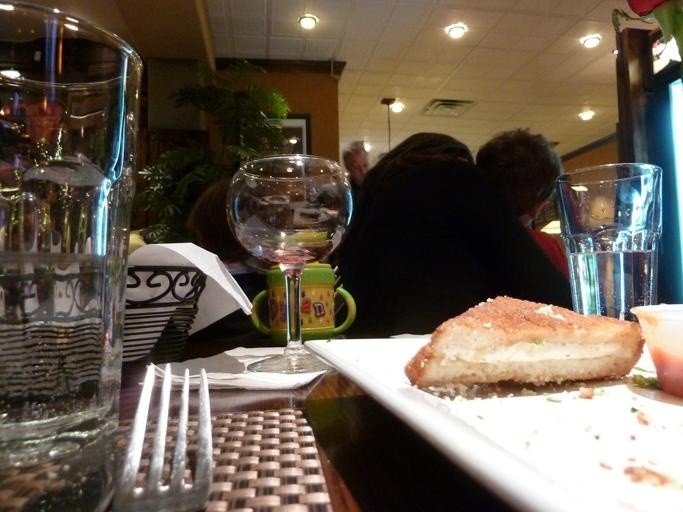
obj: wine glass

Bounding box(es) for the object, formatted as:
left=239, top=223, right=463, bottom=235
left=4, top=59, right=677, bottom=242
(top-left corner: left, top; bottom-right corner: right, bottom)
left=225, top=155, right=354, bottom=376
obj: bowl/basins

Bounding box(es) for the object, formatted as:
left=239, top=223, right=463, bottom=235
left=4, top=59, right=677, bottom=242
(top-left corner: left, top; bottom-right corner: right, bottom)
left=629, top=303, right=683, bottom=398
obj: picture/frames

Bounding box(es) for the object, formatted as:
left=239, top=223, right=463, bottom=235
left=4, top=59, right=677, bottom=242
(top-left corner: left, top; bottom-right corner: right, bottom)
left=234, top=111, right=312, bottom=172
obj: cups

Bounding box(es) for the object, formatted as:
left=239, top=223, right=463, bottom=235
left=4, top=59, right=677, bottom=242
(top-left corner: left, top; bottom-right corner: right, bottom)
left=555, top=161, right=664, bottom=319
left=248, top=262, right=357, bottom=347
left=0, top=0, right=146, bottom=512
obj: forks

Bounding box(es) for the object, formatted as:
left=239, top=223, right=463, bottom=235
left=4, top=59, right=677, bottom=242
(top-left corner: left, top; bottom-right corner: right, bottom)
left=111, top=361, right=212, bottom=511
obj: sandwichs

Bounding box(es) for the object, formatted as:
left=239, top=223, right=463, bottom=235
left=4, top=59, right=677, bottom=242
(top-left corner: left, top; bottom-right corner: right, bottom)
left=404, top=294, right=645, bottom=395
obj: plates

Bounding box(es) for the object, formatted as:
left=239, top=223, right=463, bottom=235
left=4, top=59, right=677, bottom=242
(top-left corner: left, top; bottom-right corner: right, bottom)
left=304, top=338, right=683, bottom=511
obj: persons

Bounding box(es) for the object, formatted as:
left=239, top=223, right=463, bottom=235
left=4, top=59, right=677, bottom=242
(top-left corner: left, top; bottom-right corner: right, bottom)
left=340, top=139, right=372, bottom=196
left=188, top=181, right=279, bottom=360
left=475, top=130, right=570, bottom=284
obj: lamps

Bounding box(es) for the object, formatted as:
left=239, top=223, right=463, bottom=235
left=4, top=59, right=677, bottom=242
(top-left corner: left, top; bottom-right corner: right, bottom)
left=446, top=26, right=468, bottom=38
left=390, top=98, right=405, bottom=113
left=297, top=15, right=319, bottom=29
left=582, top=36, right=601, bottom=48
left=578, top=111, right=596, bottom=121
